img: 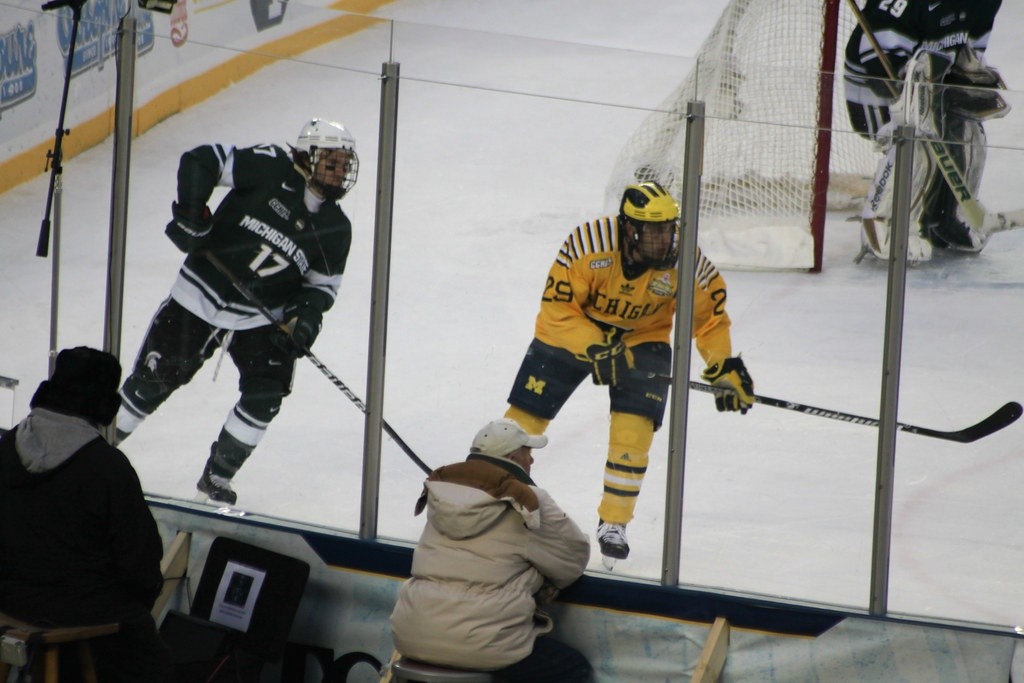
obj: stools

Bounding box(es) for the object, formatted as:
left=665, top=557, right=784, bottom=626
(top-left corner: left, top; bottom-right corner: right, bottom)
left=389, top=660, right=492, bottom=683
left=0, top=613, right=118, bottom=683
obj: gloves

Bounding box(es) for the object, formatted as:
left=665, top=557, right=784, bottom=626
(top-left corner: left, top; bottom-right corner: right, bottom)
left=576, top=328, right=636, bottom=390
left=164, top=200, right=214, bottom=256
left=269, top=300, right=323, bottom=359
left=701, top=352, right=754, bottom=414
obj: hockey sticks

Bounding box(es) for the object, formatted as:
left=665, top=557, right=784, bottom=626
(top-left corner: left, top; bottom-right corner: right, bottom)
left=628, top=368, right=1024, bottom=444
left=848, top=0, right=1024, bottom=234
left=0, top=375, right=20, bottom=390
left=202, top=249, right=436, bottom=478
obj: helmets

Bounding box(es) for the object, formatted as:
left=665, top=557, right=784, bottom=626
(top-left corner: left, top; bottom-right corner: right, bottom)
left=619, top=181, right=682, bottom=271
left=296, top=119, right=360, bottom=202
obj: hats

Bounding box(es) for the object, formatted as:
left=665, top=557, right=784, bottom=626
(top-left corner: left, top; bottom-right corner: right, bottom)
left=470, top=417, right=548, bottom=458
left=58, top=347, right=123, bottom=384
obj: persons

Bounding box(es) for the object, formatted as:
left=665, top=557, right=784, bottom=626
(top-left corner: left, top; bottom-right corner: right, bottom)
left=390, top=415, right=593, bottom=683
left=501, top=181, right=754, bottom=571
left=843, top=0, right=1012, bottom=269
left=0, top=345, right=176, bottom=683
left=112, top=117, right=359, bottom=506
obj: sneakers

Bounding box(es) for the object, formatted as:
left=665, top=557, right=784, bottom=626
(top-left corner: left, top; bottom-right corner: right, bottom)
left=596, top=518, right=630, bottom=570
left=197, top=441, right=237, bottom=507
left=917, top=212, right=984, bottom=254
left=861, top=222, right=932, bottom=264
